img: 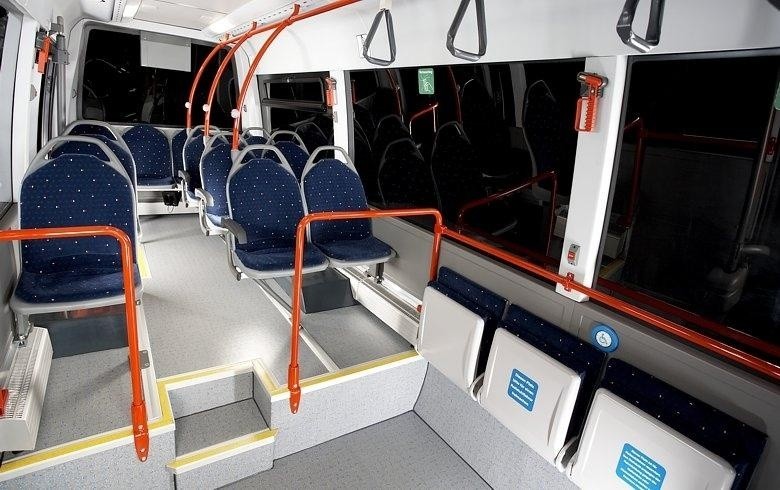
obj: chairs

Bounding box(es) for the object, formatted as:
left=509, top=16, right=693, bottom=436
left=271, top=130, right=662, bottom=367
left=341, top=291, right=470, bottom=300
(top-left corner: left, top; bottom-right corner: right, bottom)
left=122, top=123, right=180, bottom=210
left=7, top=124, right=144, bottom=347
left=568, top=357, right=768, bottom=490
left=474, top=302, right=608, bottom=472
left=170, top=128, right=399, bottom=288
left=414, top=265, right=508, bottom=400
